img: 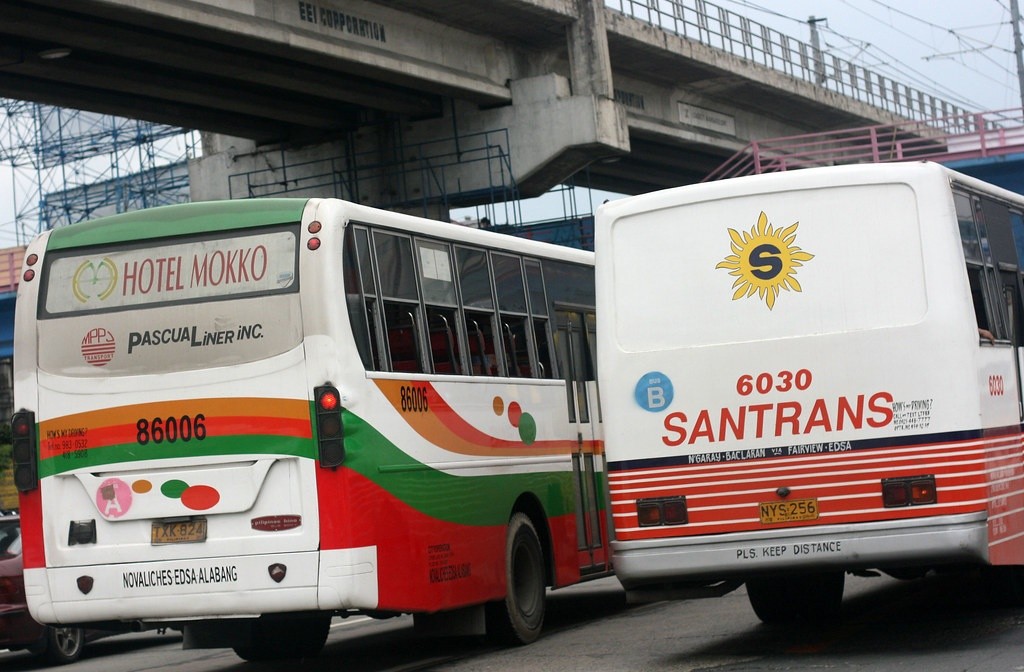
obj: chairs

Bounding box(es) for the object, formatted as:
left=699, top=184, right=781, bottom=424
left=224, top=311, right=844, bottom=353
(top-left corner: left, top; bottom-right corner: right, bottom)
left=368, top=311, right=422, bottom=373
left=466, top=319, right=492, bottom=376
left=501, top=322, right=521, bottom=377
left=427, top=314, right=458, bottom=374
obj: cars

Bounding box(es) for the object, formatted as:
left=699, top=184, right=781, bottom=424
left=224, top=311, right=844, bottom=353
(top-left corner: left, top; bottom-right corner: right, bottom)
left=0, top=516, right=88, bottom=663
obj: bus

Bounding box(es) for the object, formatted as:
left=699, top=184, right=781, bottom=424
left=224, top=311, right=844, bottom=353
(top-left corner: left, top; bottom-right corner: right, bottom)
left=14, top=197, right=596, bottom=668
left=594, top=160, right=1024, bottom=628
